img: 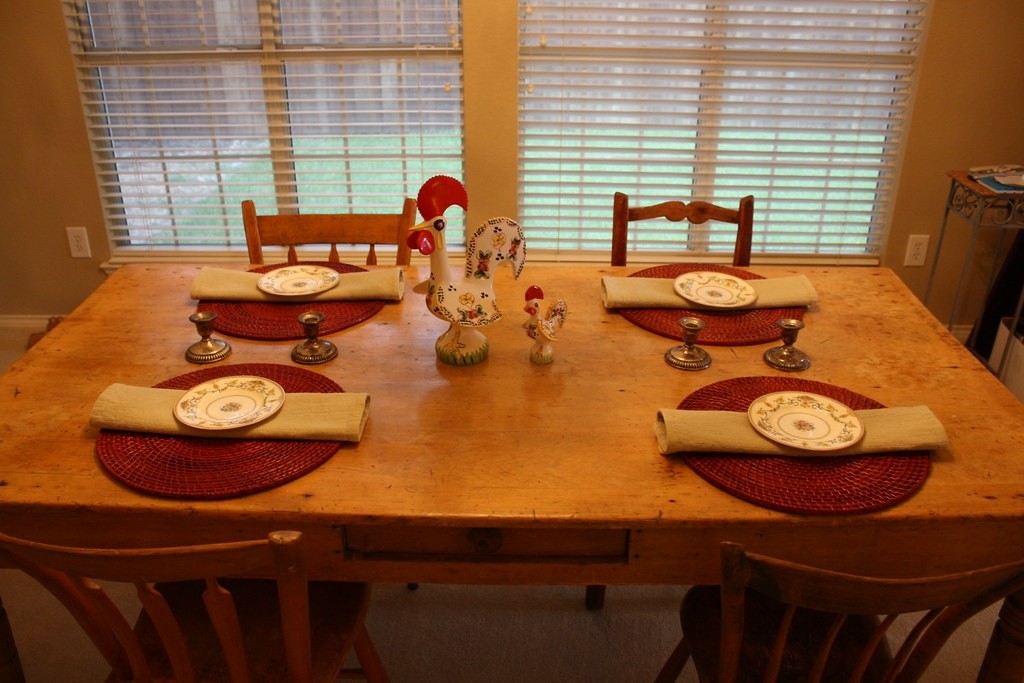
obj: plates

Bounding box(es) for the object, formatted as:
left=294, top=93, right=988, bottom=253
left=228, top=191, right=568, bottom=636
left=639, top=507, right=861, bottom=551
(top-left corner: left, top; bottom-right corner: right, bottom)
left=173, top=374, right=285, bottom=430
left=257, top=264, right=341, bottom=296
left=748, top=390, right=864, bottom=451
left=673, top=271, right=758, bottom=308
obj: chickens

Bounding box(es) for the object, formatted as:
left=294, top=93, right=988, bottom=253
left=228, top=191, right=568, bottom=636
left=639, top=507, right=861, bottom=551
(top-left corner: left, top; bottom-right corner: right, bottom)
left=523, top=285, right=568, bottom=365
left=406, top=175, right=526, bottom=367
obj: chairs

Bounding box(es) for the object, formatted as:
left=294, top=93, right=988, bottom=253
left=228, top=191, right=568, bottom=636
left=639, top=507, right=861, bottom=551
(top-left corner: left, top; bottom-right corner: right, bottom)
left=0, top=531, right=389, bottom=683
left=585, top=190, right=754, bottom=612
left=241, top=197, right=420, bottom=593
left=653, top=540, right=1024, bottom=683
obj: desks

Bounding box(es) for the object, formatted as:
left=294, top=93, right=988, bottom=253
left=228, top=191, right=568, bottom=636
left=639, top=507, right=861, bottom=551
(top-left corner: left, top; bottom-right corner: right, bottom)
left=921, top=170, right=1024, bottom=331
left=0, top=261, right=1024, bottom=683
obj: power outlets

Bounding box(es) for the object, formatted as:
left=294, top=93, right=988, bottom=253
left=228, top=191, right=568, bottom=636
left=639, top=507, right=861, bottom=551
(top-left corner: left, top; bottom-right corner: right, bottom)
left=66, top=226, right=92, bottom=258
left=903, top=234, right=930, bottom=267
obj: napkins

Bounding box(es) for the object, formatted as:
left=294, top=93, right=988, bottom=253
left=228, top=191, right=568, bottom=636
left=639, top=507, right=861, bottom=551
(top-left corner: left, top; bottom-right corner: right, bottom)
left=190, top=266, right=405, bottom=302
left=89, top=382, right=373, bottom=444
left=600, top=276, right=819, bottom=310
left=653, top=405, right=950, bottom=455
